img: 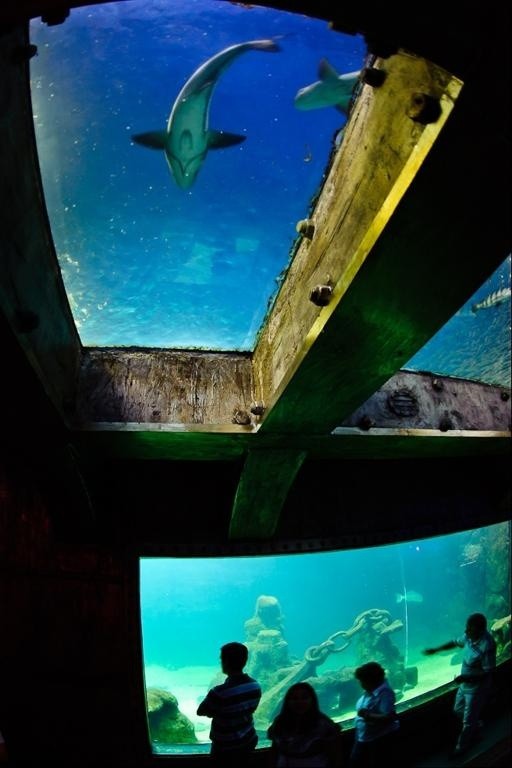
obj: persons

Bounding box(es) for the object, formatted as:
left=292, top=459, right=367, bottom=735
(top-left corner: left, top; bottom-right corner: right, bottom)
left=349, top=662, right=400, bottom=768
left=422, top=612, right=497, bottom=758
left=196, top=642, right=262, bottom=768
left=266, top=683, right=343, bottom=768
